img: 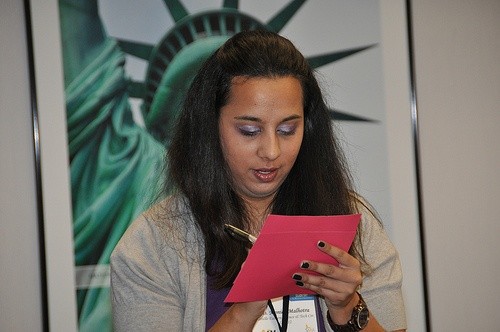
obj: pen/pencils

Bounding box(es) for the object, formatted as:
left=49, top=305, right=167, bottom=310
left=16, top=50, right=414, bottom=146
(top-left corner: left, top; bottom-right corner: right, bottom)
left=224, top=223, right=256, bottom=243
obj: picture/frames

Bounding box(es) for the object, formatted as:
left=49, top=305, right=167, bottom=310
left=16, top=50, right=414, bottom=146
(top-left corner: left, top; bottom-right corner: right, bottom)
left=23, top=0, right=432, bottom=332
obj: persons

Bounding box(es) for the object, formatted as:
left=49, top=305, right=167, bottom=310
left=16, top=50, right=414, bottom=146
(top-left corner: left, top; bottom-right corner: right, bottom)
left=107, top=32, right=407, bottom=332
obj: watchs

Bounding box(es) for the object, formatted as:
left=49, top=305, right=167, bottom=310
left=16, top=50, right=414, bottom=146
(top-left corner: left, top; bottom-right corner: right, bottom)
left=327, top=291, right=369, bottom=332
